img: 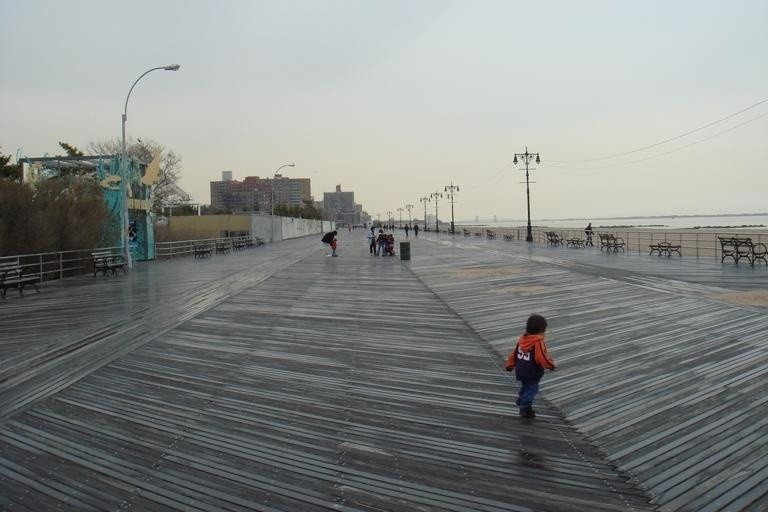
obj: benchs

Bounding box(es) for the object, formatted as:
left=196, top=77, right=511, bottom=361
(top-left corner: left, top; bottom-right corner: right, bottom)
left=545, top=231, right=626, bottom=252
left=192, top=235, right=265, bottom=259
left=0, top=261, right=41, bottom=299
left=91, top=251, right=129, bottom=277
left=717, top=236, right=768, bottom=269
left=649, top=242, right=682, bottom=258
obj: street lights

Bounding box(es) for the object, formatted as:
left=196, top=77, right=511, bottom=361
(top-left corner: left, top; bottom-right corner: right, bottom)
left=268, top=163, right=295, bottom=245
left=444, top=180, right=460, bottom=237
left=511, top=145, right=541, bottom=240
left=119, top=62, right=181, bottom=268
left=370, top=189, right=442, bottom=234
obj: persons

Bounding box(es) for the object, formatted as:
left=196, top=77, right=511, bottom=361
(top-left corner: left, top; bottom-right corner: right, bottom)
left=584, top=223, right=595, bottom=247
left=330, top=238, right=339, bottom=257
left=413, top=222, right=419, bottom=237
left=374, top=229, right=395, bottom=257
left=382, top=224, right=395, bottom=233
left=366, top=226, right=378, bottom=256
left=344, top=222, right=373, bottom=233
left=404, top=223, right=409, bottom=238
left=320, top=230, right=337, bottom=258
left=503, top=312, right=560, bottom=418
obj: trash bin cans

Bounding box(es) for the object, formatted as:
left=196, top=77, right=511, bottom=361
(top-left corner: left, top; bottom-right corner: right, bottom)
left=400, top=241, right=410, bottom=260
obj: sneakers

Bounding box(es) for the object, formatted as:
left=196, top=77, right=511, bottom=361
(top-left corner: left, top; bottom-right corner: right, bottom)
left=516, top=399, right=535, bottom=418
